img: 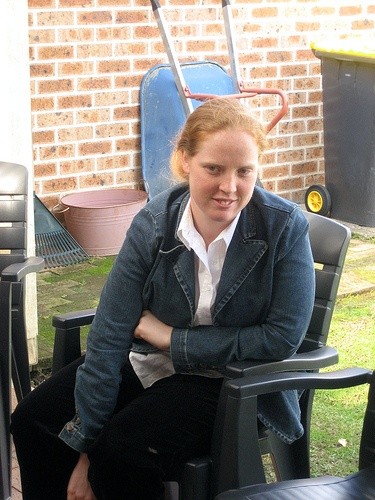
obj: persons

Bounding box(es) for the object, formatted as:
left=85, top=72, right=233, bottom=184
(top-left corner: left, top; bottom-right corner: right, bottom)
left=6, top=99, right=315, bottom=500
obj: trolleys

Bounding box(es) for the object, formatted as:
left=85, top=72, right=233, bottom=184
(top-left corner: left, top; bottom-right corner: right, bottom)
left=140, top=0, right=288, bottom=202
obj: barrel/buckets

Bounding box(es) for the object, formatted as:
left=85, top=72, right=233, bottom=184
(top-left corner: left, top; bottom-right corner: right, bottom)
left=52, top=179, right=150, bottom=256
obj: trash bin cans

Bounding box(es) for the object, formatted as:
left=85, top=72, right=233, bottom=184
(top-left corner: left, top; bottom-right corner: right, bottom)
left=303, top=40, right=375, bottom=229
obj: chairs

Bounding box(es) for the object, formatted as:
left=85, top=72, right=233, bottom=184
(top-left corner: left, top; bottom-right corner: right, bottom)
left=208, top=369, right=375, bottom=500
left=0, top=160, right=46, bottom=500
left=50, top=211, right=352, bottom=500
